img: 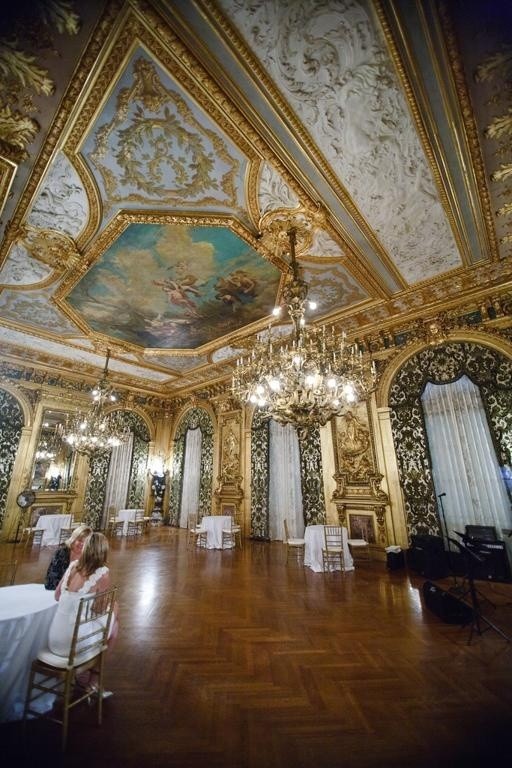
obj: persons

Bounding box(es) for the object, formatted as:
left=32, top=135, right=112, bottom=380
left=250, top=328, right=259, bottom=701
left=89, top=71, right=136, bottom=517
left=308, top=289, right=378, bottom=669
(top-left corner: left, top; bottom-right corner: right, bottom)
left=45, top=524, right=95, bottom=590
left=48, top=532, right=120, bottom=704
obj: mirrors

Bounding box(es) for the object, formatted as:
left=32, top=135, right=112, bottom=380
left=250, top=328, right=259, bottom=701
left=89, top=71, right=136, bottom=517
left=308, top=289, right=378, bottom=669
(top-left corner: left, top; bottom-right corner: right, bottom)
left=30, top=407, right=87, bottom=494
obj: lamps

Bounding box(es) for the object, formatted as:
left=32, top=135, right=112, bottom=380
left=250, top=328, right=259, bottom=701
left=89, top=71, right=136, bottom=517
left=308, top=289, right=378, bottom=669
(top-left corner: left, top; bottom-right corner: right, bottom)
left=66, top=347, right=130, bottom=461
left=229, top=228, right=378, bottom=434
left=36, top=422, right=64, bottom=465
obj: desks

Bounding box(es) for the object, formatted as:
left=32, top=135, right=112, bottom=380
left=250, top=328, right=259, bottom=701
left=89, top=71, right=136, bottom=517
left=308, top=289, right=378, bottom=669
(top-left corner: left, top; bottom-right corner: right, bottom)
left=199, top=516, right=236, bottom=551
left=0, top=582, right=60, bottom=724
left=303, top=524, right=350, bottom=572
left=34, top=513, right=75, bottom=547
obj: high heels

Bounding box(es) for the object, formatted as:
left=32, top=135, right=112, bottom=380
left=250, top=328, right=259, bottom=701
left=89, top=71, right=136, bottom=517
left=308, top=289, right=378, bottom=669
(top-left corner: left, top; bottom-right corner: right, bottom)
left=85, top=680, right=114, bottom=702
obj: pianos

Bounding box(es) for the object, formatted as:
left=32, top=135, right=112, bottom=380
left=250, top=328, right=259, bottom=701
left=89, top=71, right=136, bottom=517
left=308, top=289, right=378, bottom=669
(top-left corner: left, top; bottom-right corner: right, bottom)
left=460, top=526, right=512, bottom=581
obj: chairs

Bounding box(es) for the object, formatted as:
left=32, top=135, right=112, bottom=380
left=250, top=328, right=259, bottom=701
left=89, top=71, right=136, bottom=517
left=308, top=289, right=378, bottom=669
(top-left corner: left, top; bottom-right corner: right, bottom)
left=17, top=515, right=44, bottom=553
left=58, top=511, right=86, bottom=547
left=186, top=511, right=210, bottom=550
left=284, top=519, right=304, bottom=564
left=221, top=513, right=244, bottom=551
left=16, top=586, right=119, bottom=752
left=106, top=505, right=154, bottom=537
left=321, top=521, right=372, bottom=575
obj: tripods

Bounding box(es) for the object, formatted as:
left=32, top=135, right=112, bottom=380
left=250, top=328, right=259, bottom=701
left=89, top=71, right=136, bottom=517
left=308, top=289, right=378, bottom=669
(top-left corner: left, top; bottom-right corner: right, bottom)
left=460, top=557, right=512, bottom=648
